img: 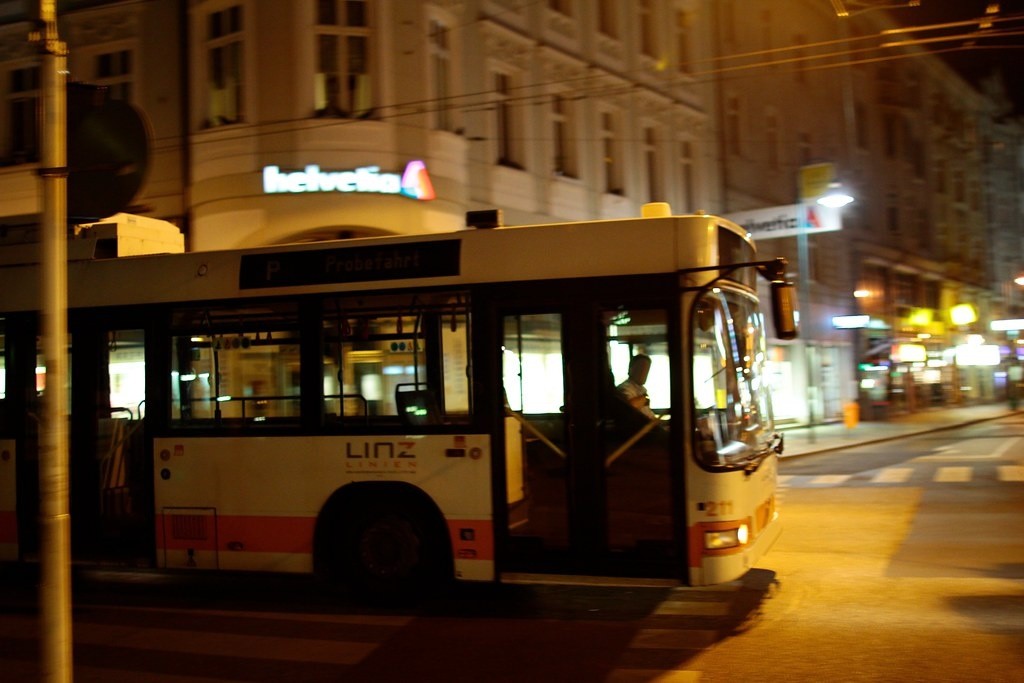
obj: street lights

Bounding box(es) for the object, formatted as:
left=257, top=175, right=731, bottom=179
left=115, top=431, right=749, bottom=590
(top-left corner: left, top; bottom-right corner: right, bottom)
left=797, top=180, right=859, bottom=443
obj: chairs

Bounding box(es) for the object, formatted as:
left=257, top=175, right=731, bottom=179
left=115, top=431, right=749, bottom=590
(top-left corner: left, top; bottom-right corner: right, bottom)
left=395, top=382, right=429, bottom=415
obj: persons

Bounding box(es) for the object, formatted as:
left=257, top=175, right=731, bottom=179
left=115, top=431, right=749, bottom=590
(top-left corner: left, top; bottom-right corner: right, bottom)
left=615, top=355, right=655, bottom=419
left=1005, top=358, right=1024, bottom=410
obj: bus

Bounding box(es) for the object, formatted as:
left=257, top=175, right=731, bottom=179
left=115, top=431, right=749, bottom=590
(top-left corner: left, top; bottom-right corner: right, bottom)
left=0, top=203, right=800, bottom=613
